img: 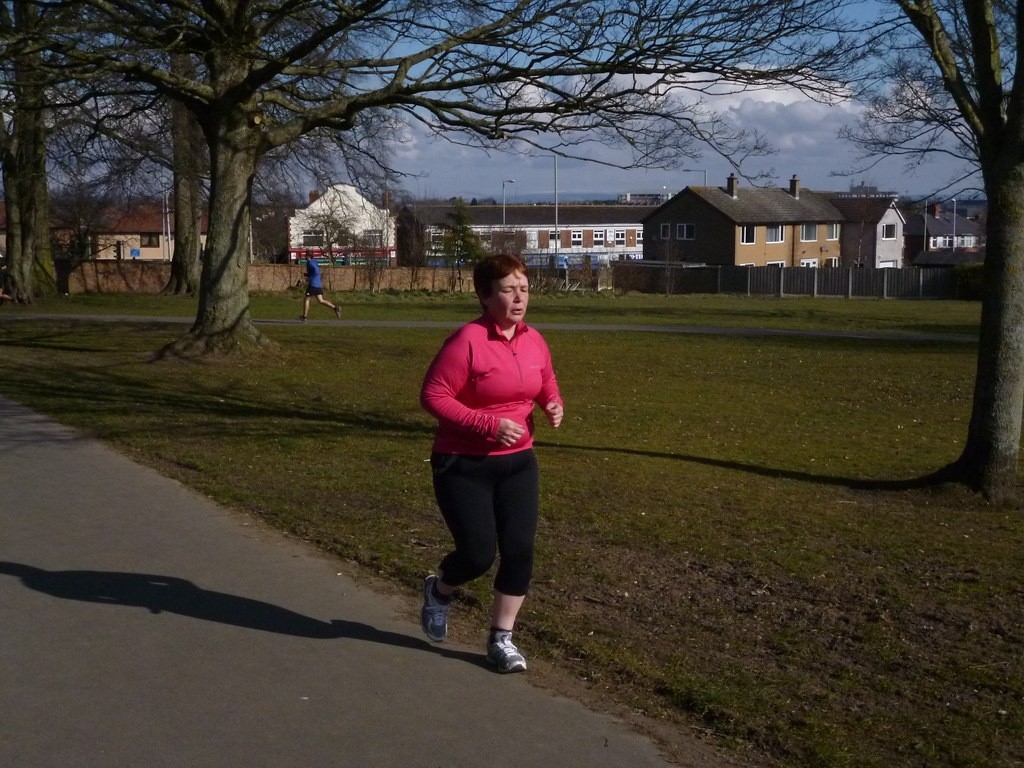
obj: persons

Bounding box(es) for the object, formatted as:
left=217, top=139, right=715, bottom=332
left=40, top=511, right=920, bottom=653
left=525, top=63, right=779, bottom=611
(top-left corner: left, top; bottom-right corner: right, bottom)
left=420, top=254, right=564, bottom=673
left=298, top=250, right=341, bottom=321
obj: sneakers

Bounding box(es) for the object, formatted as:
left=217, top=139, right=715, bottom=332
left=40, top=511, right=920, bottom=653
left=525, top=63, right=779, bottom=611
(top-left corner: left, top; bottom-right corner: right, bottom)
left=336, top=306, right=342, bottom=319
left=420, top=574, right=450, bottom=642
left=296, top=315, right=308, bottom=322
left=487, top=632, right=528, bottom=674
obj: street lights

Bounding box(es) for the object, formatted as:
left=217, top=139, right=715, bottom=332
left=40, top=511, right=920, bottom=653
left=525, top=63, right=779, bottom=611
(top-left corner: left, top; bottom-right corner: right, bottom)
left=386, top=190, right=399, bottom=268
left=528, top=154, right=558, bottom=276
left=165, top=189, right=175, bottom=262
left=951, top=198, right=956, bottom=251
left=683, top=169, right=707, bottom=187
left=502, top=179, right=516, bottom=231
